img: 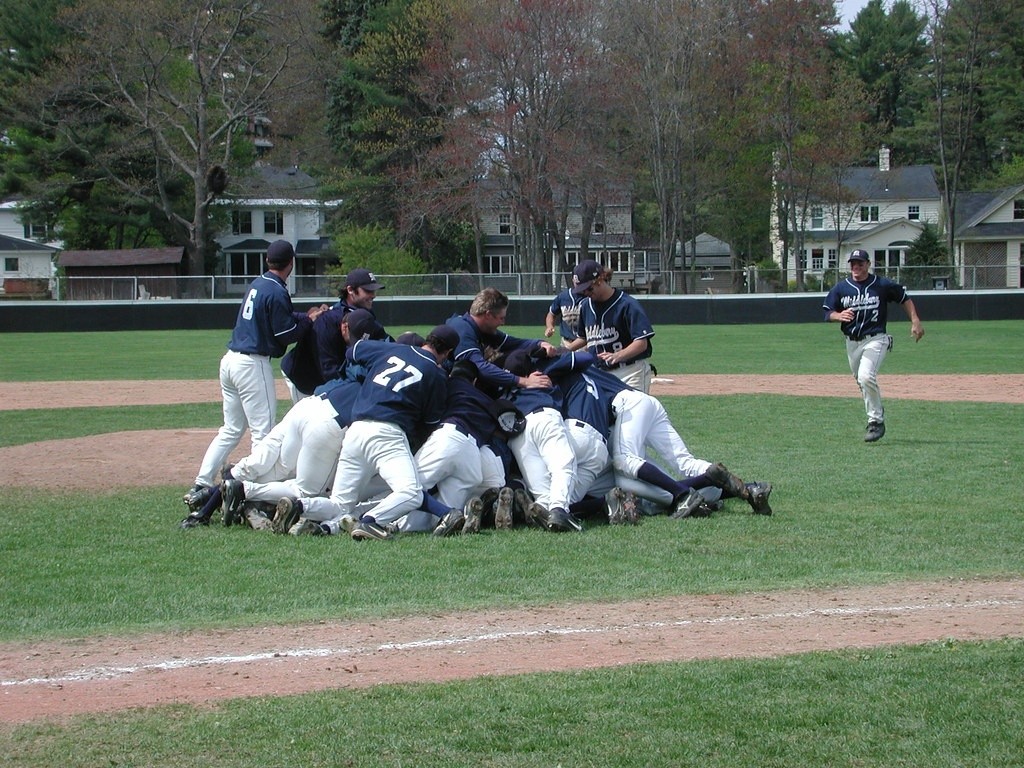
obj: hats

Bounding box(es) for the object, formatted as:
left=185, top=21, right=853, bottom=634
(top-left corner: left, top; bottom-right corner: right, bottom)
left=574, top=260, right=599, bottom=292
left=432, top=324, right=460, bottom=353
left=397, top=332, right=425, bottom=345
left=346, top=269, right=385, bottom=290
left=488, top=399, right=526, bottom=434
left=848, top=249, right=869, bottom=263
left=268, top=240, right=296, bottom=262
left=349, top=308, right=374, bottom=345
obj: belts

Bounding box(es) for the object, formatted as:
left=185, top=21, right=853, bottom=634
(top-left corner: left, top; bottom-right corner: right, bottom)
left=487, top=446, right=501, bottom=456
left=606, top=360, right=635, bottom=371
left=576, top=422, right=607, bottom=444
left=434, top=424, right=481, bottom=449
left=241, top=352, right=250, bottom=355
left=533, top=407, right=543, bottom=414
left=564, top=337, right=574, bottom=342
left=321, top=393, right=346, bottom=429
left=850, top=333, right=877, bottom=340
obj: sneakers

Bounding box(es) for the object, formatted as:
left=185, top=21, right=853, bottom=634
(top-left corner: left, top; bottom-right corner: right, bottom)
left=181, top=461, right=773, bottom=541
left=864, top=421, right=885, bottom=441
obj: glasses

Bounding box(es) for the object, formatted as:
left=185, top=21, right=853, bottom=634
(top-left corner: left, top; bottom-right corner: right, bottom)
left=585, top=281, right=598, bottom=291
left=851, top=261, right=864, bottom=266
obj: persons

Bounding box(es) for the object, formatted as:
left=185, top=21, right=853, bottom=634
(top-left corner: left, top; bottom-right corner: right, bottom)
left=822, top=248, right=925, bottom=442
left=183, top=238, right=328, bottom=513
left=545, top=280, right=582, bottom=344
left=444, top=286, right=556, bottom=389
left=560, top=261, right=656, bottom=396
left=182, top=306, right=773, bottom=541
left=279, top=268, right=385, bottom=402
